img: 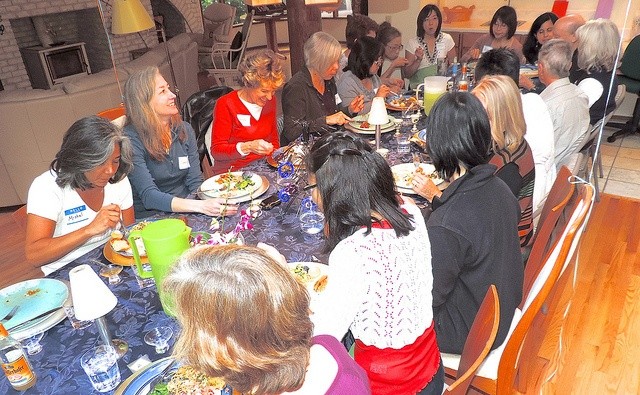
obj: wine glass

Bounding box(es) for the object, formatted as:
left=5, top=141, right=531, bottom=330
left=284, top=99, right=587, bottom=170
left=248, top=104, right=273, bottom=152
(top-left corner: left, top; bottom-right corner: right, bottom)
left=143, top=327, right=173, bottom=355
left=19, top=329, right=44, bottom=356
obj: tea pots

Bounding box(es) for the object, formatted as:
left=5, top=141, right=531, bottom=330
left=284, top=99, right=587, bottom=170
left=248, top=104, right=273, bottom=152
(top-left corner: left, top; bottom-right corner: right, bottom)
left=416, top=76, right=452, bottom=117
left=129, top=218, right=192, bottom=321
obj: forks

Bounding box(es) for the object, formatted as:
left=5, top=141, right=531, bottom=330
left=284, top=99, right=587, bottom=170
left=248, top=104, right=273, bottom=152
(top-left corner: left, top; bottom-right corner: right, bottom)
left=413, top=154, right=420, bottom=172
left=0, top=304, right=23, bottom=325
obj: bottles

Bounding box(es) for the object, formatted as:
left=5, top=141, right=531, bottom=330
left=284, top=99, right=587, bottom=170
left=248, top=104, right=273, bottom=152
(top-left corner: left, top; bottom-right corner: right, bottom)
left=457, top=63, right=469, bottom=91
left=449, top=57, right=460, bottom=90
left=1, top=323, right=35, bottom=392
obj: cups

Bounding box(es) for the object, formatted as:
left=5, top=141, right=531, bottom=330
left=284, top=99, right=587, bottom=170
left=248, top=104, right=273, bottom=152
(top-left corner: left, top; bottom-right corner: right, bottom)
left=397, top=133, right=411, bottom=154
left=437, top=54, right=450, bottom=76
left=300, top=212, right=325, bottom=241
left=80, top=345, right=122, bottom=393
left=402, top=108, right=413, bottom=126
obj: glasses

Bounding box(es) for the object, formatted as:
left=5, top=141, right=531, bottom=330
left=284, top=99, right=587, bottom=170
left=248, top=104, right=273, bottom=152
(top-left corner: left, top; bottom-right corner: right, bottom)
left=374, top=59, right=383, bottom=65
left=388, top=44, right=403, bottom=51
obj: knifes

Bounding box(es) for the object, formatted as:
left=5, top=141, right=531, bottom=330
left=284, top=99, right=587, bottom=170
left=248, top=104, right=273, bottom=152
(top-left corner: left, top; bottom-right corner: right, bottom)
left=7, top=305, right=63, bottom=333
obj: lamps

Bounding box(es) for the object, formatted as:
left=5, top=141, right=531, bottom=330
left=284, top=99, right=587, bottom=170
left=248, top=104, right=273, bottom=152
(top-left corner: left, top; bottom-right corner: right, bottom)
left=369, top=97, right=391, bottom=155
left=68, top=264, right=129, bottom=360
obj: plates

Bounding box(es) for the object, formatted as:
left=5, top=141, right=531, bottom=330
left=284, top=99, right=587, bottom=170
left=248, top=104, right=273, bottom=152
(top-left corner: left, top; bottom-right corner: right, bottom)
left=201, top=171, right=262, bottom=198
left=390, top=162, right=445, bottom=189
left=266, top=145, right=309, bottom=167
left=0, top=279, right=74, bottom=341
left=349, top=116, right=395, bottom=131
left=198, top=168, right=268, bottom=204
left=394, top=181, right=451, bottom=194
left=288, top=262, right=330, bottom=311
left=116, top=357, right=240, bottom=395
left=386, top=103, right=417, bottom=111
left=344, top=113, right=397, bottom=135
left=104, top=237, right=148, bottom=267
left=0, top=278, right=68, bottom=335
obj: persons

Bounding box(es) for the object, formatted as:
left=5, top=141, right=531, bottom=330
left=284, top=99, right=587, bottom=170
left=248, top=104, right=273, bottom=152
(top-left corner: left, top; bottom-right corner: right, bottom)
left=408, top=91, right=524, bottom=354
left=400, top=3, right=459, bottom=91
left=475, top=45, right=558, bottom=235
left=536, top=38, right=590, bottom=175
left=378, top=21, right=410, bottom=90
left=337, top=35, right=403, bottom=115
left=122, top=64, right=238, bottom=223
left=516, top=15, right=590, bottom=94
left=522, top=13, right=560, bottom=66
left=160, top=245, right=371, bottom=395
left=210, top=49, right=286, bottom=175
left=458, top=5, right=526, bottom=65
left=334, top=11, right=404, bottom=88
left=301, top=131, right=445, bottom=395
left=571, top=18, right=623, bottom=127
left=24, top=116, right=134, bottom=278
left=411, top=76, right=534, bottom=265
left=280, top=31, right=365, bottom=147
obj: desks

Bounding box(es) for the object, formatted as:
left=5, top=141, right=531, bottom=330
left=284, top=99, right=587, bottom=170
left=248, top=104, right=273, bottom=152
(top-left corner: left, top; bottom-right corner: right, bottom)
left=440, top=17, right=532, bottom=62
left=250, top=5, right=290, bottom=59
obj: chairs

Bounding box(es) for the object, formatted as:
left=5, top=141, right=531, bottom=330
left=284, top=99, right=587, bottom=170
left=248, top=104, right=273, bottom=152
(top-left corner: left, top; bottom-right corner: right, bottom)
left=605, top=35, right=636, bottom=142
left=189, top=3, right=235, bottom=67
left=185, top=87, right=235, bottom=169
left=207, top=11, right=255, bottom=90
left=445, top=285, right=500, bottom=395
left=586, top=82, right=626, bottom=201
left=438, top=167, right=596, bottom=392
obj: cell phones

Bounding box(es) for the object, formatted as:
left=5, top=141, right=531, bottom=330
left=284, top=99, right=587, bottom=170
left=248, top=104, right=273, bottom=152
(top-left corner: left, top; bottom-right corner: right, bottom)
left=259, top=193, right=281, bottom=211
